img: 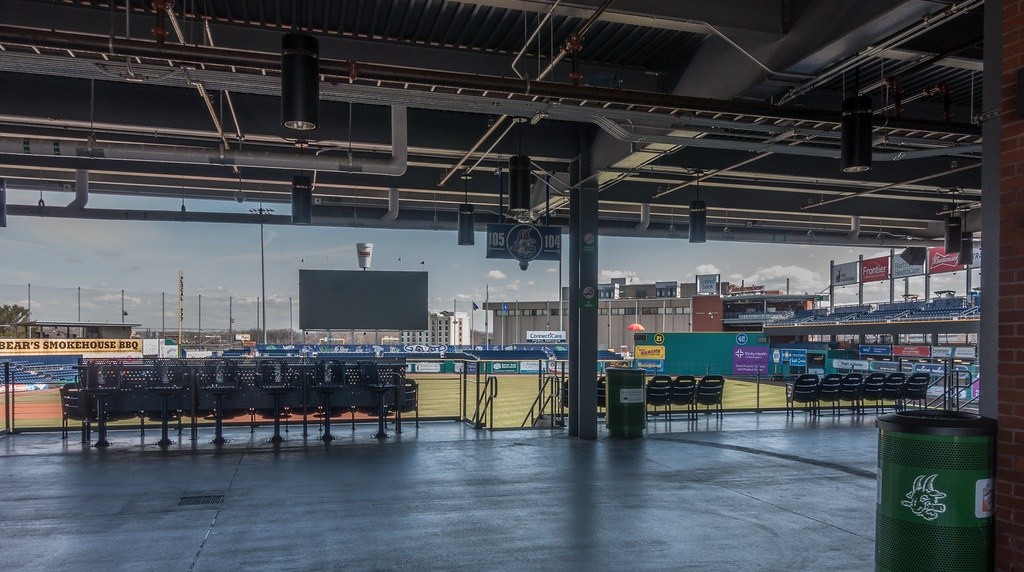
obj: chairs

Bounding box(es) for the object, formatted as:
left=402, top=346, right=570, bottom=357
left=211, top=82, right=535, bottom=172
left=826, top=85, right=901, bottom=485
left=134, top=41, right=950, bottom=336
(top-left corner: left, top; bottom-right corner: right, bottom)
left=558, top=379, right=569, bottom=420
left=769, top=305, right=979, bottom=326
left=597, top=377, right=607, bottom=407
left=0, top=353, right=418, bottom=447
left=646, top=374, right=725, bottom=421
left=787, top=373, right=930, bottom=417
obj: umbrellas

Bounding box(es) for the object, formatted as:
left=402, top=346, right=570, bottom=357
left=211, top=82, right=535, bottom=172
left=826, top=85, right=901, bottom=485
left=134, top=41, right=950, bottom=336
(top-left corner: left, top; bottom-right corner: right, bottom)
left=625, top=323, right=645, bottom=333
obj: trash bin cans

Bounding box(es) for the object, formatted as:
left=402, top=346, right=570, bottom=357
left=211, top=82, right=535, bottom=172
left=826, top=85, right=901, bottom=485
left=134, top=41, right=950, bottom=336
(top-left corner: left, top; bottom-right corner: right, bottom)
left=604, top=366, right=646, bottom=438
left=874, top=409, right=997, bottom=572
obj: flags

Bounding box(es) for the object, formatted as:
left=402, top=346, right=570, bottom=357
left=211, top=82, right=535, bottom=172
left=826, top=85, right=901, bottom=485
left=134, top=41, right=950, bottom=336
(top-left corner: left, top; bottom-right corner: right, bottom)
left=473, top=302, right=478, bottom=311
left=503, top=303, right=508, bottom=312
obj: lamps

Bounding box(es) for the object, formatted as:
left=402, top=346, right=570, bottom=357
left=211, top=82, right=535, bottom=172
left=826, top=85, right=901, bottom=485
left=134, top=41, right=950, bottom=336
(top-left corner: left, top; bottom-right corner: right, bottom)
left=689, top=171, right=706, bottom=243
left=458, top=168, right=475, bottom=245
left=283, top=0, right=319, bottom=131
left=0, top=179, right=6, bottom=228
left=840, top=51, right=872, bottom=173
left=958, top=207, right=973, bottom=264
left=945, top=169, right=961, bottom=255
left=509, top=116, right=530, bottom=211
left=292, top=133, right=311, bottom=225
